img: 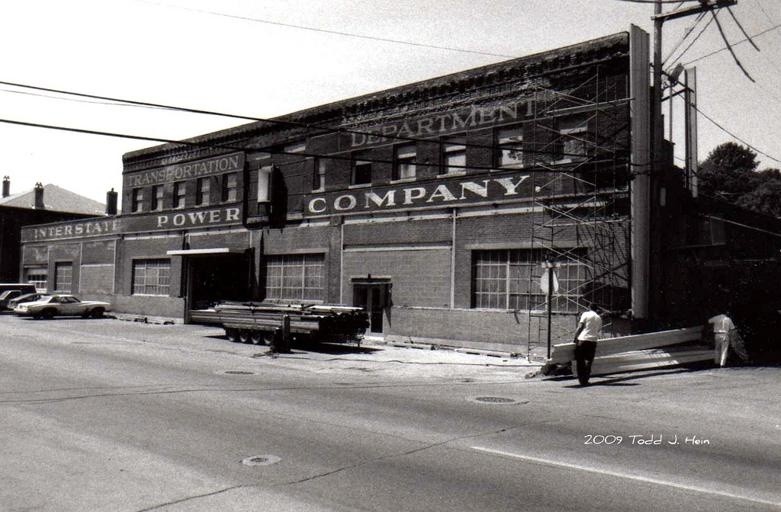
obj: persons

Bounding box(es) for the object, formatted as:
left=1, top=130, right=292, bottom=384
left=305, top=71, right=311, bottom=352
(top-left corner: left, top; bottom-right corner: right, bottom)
left=573, top=300, right=604, bottom=386
left=709, top=307, right=737, bottom=370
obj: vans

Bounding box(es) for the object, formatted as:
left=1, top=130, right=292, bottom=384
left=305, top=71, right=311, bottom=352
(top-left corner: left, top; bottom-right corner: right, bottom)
left=0, top=282, right=37, bottom=311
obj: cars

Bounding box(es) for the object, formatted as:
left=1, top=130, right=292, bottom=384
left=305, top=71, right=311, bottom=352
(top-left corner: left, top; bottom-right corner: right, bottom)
left=7, top=292, right=51, bottom=310
left=14, top=295, right=112, bottom=319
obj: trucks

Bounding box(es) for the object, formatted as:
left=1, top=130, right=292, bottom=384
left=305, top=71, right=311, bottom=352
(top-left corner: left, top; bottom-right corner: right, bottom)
left=1, top=290, right=30, bottom=310
